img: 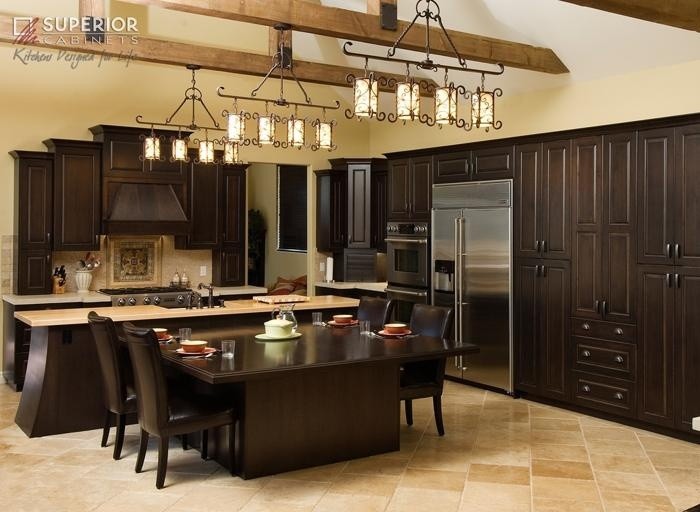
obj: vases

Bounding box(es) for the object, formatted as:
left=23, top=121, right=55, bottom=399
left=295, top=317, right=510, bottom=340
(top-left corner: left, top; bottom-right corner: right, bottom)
left=75, top=270, right=93, bottom=293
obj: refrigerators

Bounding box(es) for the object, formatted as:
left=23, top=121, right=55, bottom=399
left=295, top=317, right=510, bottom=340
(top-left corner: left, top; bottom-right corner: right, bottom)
left=432, top=179, right=512, bottom=396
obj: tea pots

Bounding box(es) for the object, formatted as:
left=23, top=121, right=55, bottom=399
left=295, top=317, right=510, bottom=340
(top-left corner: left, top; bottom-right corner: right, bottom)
left=272, top=304, right=298, bottom=330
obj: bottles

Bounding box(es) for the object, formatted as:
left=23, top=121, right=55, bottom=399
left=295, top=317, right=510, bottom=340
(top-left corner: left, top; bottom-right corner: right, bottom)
left=172, top=272, right=180, bottom=286
left=180, top=272, right=188, bottom=285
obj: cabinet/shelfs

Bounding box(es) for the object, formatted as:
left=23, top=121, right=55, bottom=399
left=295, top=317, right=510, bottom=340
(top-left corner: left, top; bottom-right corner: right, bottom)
left=224, top=169, right=245, bottom=247
left=12, top=249, right=52, bottom=295
left=174, top=154, right=226, bottom=251
left=1, top=299, right=113, bottom=392
left=248, top=228, right=266, bottom=287
left=93, top=132, right=187, bottom=178
left=13, top=158, right=53, bottom=250
left=48, top=146, right=102, bottom=252
left=212, top=247, right=246, bottom=287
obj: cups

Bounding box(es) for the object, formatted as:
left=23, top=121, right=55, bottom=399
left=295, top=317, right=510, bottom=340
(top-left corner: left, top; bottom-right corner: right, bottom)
left=359, top=320, right=370, bottom=334
left=221, top=339, right=236, bottom=359
left=312, top=312, right=322, bottom=327
left=178, top=328, right=191, bottom=341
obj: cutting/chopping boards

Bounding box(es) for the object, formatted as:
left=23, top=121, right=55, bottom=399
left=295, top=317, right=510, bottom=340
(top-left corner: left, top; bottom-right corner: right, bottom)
left=253, top=294, right=310, bottom=303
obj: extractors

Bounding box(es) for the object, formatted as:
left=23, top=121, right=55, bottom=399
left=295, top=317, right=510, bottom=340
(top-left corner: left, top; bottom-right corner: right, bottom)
left=104, top=184, right=188, bottom=236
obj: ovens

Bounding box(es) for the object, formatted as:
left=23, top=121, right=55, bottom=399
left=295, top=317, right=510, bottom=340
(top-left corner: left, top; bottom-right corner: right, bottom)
left=385, top=235, right=430, bottom=325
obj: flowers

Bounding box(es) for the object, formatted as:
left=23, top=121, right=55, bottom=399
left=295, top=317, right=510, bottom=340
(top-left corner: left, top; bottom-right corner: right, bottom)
left=76, top=252, right=104, bottom=271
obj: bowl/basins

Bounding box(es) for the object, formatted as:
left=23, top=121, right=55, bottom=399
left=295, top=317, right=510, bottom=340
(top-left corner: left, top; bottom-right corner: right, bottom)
left=264, top=317, right=295, bottom=337
left=332, top=315, right=353, bottom=323
left=152, top=328, right=168, bottom=337
left=179, top=339, right=207, bottom=352
left=384, top=323, right=407, bottom=333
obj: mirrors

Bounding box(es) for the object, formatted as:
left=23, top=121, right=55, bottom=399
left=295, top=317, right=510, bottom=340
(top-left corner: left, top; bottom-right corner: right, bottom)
left=275, top=164, right=307, bottom=253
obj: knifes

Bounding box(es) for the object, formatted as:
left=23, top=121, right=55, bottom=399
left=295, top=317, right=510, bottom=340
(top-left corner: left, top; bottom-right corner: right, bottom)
left=54, top=264, right=67, bottom=286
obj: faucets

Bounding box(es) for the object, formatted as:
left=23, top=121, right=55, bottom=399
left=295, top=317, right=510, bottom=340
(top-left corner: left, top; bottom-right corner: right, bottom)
left=197, top=282, right=215, bottom=308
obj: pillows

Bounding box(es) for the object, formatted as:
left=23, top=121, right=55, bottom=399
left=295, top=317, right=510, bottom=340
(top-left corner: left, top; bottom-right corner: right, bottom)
left=269, top=275, right=307, bottom=296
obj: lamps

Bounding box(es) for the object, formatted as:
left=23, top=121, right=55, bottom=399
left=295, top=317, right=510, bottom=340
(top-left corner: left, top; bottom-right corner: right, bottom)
left=216, top=22, right=340, bottom=152
left=341, top=0, right=504, bottom=132
left=135, top=64, right=242, bottom=167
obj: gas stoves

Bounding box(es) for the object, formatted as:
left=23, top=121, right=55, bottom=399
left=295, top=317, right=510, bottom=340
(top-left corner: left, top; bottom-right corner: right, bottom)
left=98, top=286, right=195, bottom=310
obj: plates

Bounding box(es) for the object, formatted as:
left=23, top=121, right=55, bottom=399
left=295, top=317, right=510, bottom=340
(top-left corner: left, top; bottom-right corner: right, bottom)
left=327, top=320, right=358, bottom=326
left=156, top=335, right=172, bottom=341
left=379, top=329, right=412, bottom=336
left=176, top=347, right=216, bottom=355
left=255, top=333, right=302, bottom=340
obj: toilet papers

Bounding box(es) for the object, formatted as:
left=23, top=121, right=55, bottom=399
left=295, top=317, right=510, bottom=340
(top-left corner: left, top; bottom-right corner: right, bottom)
left=325, top=256, right=335, bottom=280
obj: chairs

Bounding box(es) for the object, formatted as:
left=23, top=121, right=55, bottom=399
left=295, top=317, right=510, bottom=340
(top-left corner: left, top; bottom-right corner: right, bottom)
left=88, top=311, right=188, bottom=460
left=121, top=321, right=241, bottom=490
